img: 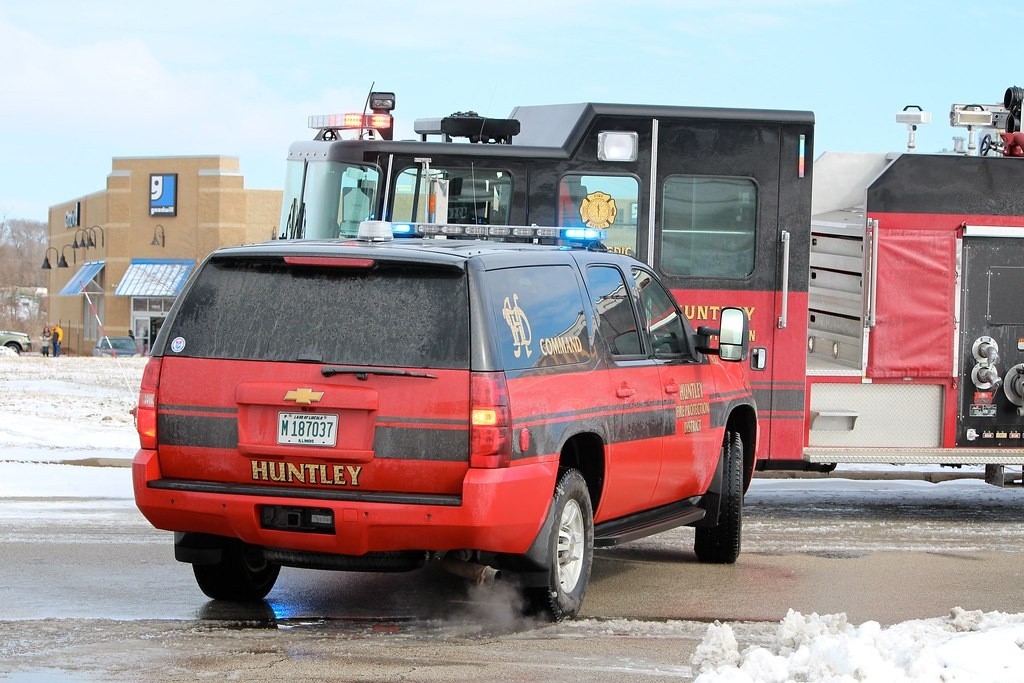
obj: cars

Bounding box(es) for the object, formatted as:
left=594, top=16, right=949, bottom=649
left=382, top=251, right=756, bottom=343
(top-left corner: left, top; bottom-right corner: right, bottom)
left=0, top=330, right=31, bottom=355
left=92, top=336, right=142, bottom=359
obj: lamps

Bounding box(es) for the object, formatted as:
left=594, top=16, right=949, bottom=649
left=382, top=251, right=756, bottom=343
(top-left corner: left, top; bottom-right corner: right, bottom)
left=72, top=230, right=82, bottom=248
left=57, top=244, right=76, bottom=267
left=79, top=228, right=97, bottom=248
left=151, top=224, right=166, bottom=247
left=40, top=247, right=59, bottom=269
left=84, top=225, right=104, bottom=248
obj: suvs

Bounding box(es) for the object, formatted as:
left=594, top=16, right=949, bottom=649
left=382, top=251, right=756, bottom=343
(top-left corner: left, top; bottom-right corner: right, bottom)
left=129, top=221, right=761, bottom=625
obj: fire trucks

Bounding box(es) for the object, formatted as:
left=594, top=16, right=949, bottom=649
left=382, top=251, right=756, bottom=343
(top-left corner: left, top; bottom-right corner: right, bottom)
left=277, top=79, right=1023, bottom=492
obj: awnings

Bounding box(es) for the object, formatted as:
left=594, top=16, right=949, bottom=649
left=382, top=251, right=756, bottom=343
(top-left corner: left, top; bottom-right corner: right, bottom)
left=59, top=260, right=104, bottom=296
left=112, top=258, right=196, bottom=297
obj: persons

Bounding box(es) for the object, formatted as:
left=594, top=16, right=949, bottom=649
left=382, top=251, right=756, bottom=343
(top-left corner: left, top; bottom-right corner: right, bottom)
left=49, top=323, right=63, bottom=357
left=128, top=329, right=135, bottom=340
left=143, top=330, right=148, bottom=352
left=40, top=326, right=51, bottom=357
